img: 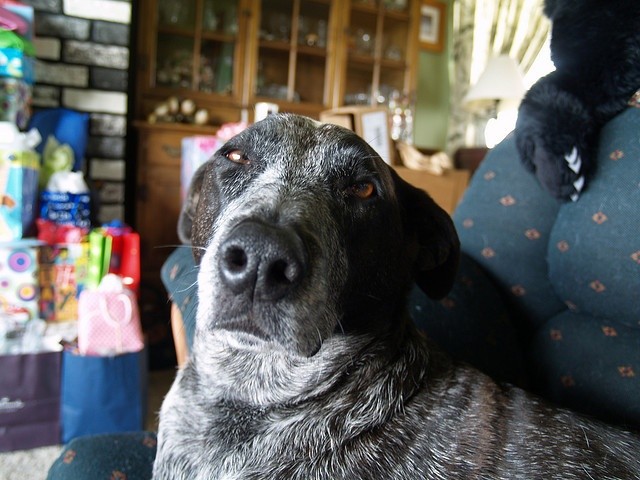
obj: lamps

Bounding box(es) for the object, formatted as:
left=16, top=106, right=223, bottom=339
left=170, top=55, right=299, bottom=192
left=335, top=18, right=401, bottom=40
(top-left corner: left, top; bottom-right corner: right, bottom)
left=462, top=56, right=528, bottom=120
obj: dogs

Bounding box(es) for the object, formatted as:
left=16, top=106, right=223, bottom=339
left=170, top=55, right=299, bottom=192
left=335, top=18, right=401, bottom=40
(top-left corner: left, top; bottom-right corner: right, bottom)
left=151, top=113, right=640, bottom=480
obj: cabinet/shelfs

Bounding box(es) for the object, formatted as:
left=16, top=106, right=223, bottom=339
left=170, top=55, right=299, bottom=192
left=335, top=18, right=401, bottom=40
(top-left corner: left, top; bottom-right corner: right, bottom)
left=335, top=0, right=421, bottom=110
left=131, top=0, right=248, bottom=346
left=242, top=0, right=342, bottom=124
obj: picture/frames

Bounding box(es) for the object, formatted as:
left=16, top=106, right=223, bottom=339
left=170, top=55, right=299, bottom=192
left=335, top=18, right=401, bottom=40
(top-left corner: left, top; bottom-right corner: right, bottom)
left=419, top=0, right=447, bottom=52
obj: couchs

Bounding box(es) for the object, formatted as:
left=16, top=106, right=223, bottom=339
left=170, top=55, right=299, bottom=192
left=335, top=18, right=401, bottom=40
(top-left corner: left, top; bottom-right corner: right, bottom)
left=47, top=106, right=640, bottom=478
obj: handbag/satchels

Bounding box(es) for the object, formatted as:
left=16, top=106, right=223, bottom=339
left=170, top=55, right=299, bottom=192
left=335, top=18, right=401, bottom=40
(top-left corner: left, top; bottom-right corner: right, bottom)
left=30, top=108, right=91, bottom=187
left=105, top=227, right=141, bottom=299
left=0, top=337, right=65, bottom=454
left=60, top=347, right=144, bottom=446
left=87, top=229, right=113, bottom=287
left=0, top=147, right=43, bottom=240
left=77, top=287, right=146, bottom=358
